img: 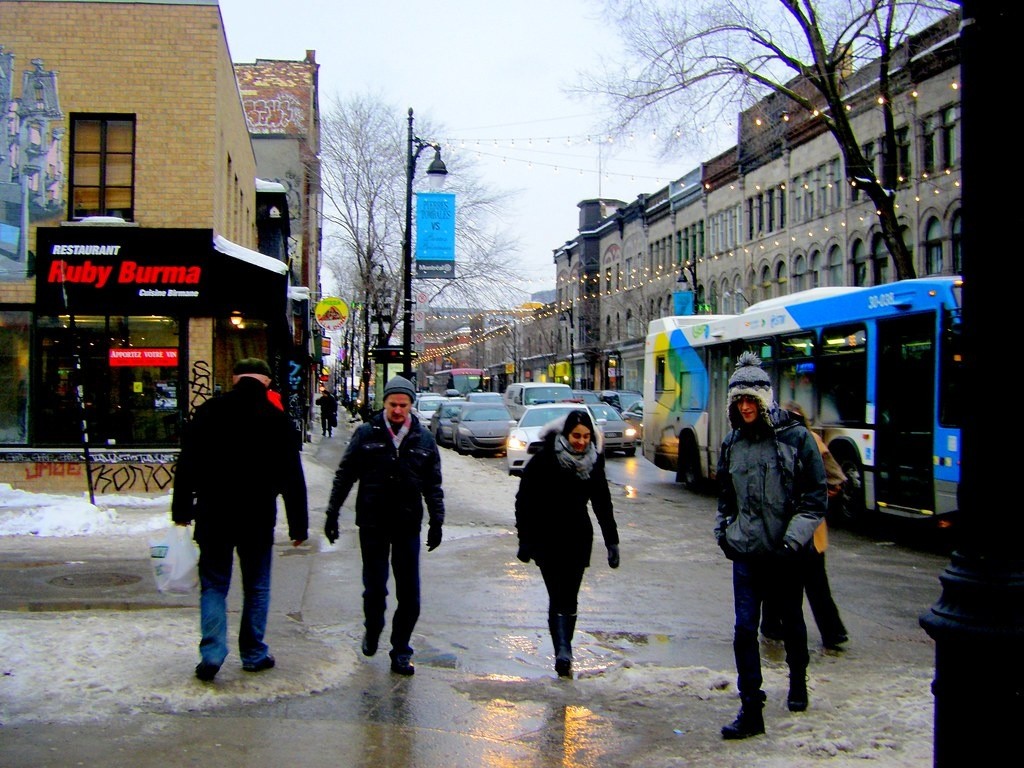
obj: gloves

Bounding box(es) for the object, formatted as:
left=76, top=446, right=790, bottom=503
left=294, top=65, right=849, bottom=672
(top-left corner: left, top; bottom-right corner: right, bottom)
left=516, top=548, right=531, bottom=564
left=324, top=516, right=340, bottom=544
left=426, top=527, right=442, bottom=553
left=606, top=545, right=620, bottom=568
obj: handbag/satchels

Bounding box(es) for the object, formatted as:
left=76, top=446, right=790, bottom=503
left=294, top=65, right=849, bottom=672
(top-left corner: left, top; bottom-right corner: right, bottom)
left=149, top=525, right=197, bottom=597
left=332, top=413, right=337, bottom=427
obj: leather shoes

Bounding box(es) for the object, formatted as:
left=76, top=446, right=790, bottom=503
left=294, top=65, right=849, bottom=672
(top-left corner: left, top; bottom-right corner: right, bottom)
left=391, top=657, right=414, bottom=675
left=361, top=630, right=378, bottom=656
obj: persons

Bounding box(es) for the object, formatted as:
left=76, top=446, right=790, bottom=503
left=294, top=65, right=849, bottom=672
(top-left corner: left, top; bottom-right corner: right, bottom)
left=324, top=375, right=445, bottom=675
left=261, top=383, right=374, bottom=438
left=515, top=410, right=620, bottom=674
left=714, top=354, right=829, bottom=738
left=172, top=359, right=309, bottom=682
left=760, top=341, right=933, bottom=645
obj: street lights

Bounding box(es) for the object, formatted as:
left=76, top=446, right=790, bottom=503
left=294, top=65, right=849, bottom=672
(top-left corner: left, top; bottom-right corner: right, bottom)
left=403, top=107, right=448, bottom=410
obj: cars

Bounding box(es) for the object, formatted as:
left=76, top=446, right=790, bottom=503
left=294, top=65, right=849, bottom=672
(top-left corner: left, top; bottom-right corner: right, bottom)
left=412, top=382, right=643, bottom=474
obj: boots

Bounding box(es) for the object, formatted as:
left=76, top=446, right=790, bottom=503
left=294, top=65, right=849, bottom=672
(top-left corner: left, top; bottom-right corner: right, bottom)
left=547, top=609, right=581, bottom=676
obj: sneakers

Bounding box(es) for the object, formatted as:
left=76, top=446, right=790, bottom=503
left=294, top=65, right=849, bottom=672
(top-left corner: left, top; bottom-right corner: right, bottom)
left=786, top=671, right=811, bottom=712
left=720, top=709, right=765, bottom=739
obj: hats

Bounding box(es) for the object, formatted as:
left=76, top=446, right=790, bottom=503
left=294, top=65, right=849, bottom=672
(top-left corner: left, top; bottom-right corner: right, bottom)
left=726, top=351, right=779, bottom=429
left=383, top=376, right=417, bottom=403
left=233, top=358, right=272, bottom=379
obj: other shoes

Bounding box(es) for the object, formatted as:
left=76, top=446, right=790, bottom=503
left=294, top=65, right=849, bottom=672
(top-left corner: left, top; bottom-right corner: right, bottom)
left=195, top=660, right=220, bottom=682
left=243, top=655, right=275, bottom=673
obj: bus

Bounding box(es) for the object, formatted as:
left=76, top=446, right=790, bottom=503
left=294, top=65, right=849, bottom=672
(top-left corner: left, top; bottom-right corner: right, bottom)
left=638, top=274, right=965, bottom=528
left=432, top=368, right=489, bottom=394
